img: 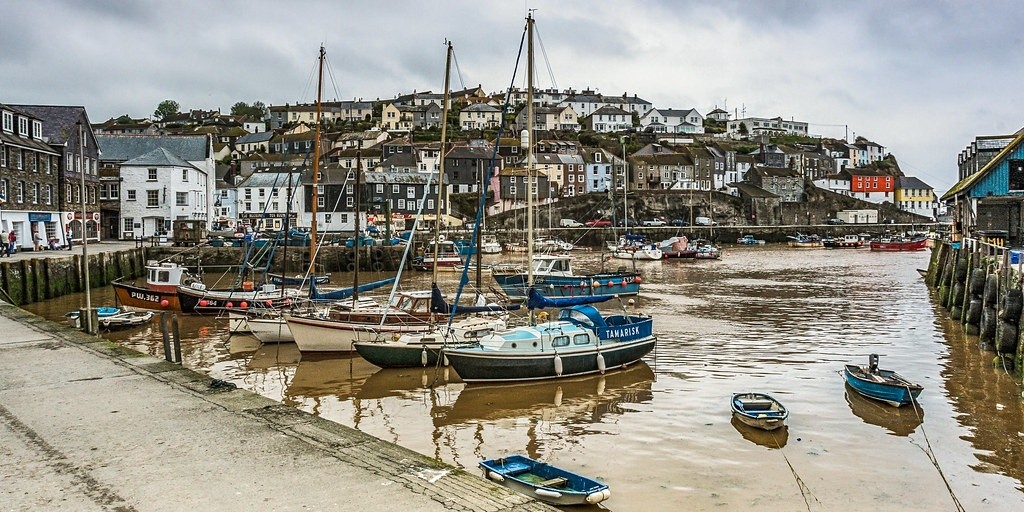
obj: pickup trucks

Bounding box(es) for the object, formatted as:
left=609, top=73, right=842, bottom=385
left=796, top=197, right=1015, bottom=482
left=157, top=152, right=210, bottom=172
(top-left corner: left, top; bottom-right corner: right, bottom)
left=639, top=217, right=667, bottom=227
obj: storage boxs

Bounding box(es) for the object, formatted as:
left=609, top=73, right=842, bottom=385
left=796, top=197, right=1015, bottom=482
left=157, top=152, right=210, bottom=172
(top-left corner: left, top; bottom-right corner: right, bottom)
left=1010, top=249, right=1024, bottom=264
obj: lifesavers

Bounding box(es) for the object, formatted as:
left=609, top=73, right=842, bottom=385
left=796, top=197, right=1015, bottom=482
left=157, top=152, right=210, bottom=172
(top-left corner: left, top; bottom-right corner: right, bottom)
left=302, top=250, right=311, bottom=273
left=964, top=324, right=975, bottom=335
left=934, top=288, right=948, bottom=304
left=940, top=264, right=953, bottom=285
left=373, top=262, right=384, bottom=271
left=954, top=257, right=968, bottom=281
left=983, top=274, right=998, bottom=301
left=991, top=356, right=1013, bottom=372
left=949, top=306, right=961, bottom=319
left=347, top=262, right=356, bottom=272
left=968, top=268, right=984, bottom=295
left=978, top=341, right=992, bottom=351
left=372, top=249, right=384, bottom=260
left=993, top=320, right=1017, bottom=352
left=949, top=284, right=964, bottom=305
left=1000, top=295, right=1023, bottom=323
left=538, top=311, right=549, bottom=324
left=979, top=307, right=995, bottom=338
left=963, top=297, right=980, bottom=323
left=347, top=251, right=358, bottom=261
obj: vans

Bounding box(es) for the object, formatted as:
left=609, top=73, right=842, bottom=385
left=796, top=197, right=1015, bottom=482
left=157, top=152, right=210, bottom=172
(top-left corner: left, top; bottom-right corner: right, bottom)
left=559, top=219, right=584, bottom=228
left=694, top=216, right=718, bottom=226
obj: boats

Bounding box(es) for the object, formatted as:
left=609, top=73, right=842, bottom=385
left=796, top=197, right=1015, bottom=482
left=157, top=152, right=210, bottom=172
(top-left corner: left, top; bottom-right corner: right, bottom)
left=109, top=238, right=212, bottom=311
left=242, top=340, right=301, bottom=378
left=736, top=235, right=766, bottom=246
left=842, top=353, right=924, bottom=412
left=439, top=362, right=658, bottom=456
left=347, top=362, right=459, bottom=407
left=63, top=307, right=122, bottom=328
left=477, top=454, right=611, bottom=505
left=99, top=311, right=156, bottom=328
left=729, top=416, right=790, bottom=449
left=869, top=216, right=928, bottom=251
left=225, top=332, right=261, bottom=362
left=820, top=233, right=863, bottom=249
left=842, top=380, right=925, bottom=441
left=281, top=349, right=383, bottom=406
left=731, top=391, right=789, bottom=432
left=787, top=233, right=825, bottom=248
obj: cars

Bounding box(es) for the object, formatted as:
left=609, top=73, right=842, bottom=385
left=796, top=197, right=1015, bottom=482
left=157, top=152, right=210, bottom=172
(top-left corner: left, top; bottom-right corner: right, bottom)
left=669, top=218, right=690, bottom=227
left=613, top=218, right=638, bottom=227
left=828, top=218, right=846, bottom=225
left=584, top=220, right=612, bottom=228
left=883, top=217, right=902, bottom=225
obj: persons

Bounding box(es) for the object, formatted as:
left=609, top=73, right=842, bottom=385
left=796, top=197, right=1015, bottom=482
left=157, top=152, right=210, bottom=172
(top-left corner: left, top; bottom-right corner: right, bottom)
left=9, top=231, right=16, bottom=254
left=154, top=229, right=160, bottom=246
left=34, top=231, right=43, bottom=251
left=0, top=229, right=10, bottom=258
left=66, top=227, right=73, bottom=250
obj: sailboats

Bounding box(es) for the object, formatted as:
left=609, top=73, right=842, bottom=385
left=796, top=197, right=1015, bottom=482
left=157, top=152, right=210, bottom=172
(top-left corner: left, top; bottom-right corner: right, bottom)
left=223, top=8, right=725, bottom=383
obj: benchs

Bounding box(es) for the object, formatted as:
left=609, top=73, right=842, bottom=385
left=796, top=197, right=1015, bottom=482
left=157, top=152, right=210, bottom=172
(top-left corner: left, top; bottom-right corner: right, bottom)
left=539, top=476, right=568, bottom=487
left=738, top=399, right=772, bottom=406
left=746, top=410, right=784, bottom=415
left=491, top=460, right=531, bottom=476
